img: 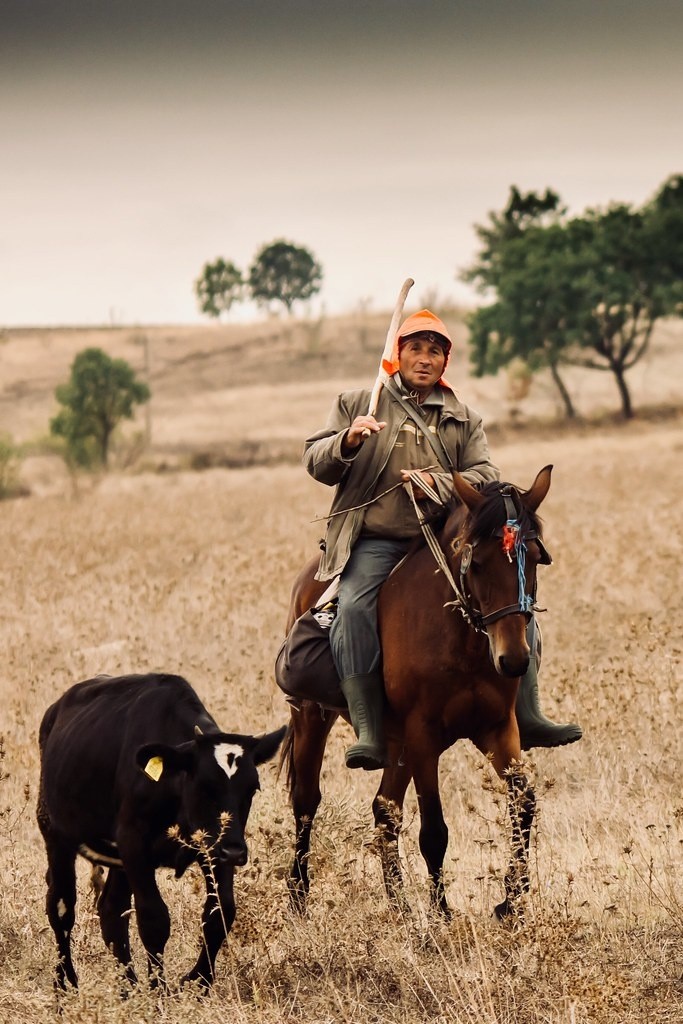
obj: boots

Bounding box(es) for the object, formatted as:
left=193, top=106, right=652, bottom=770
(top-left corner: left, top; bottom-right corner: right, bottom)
left=515, top=656, right=583, bottom=752
left=339, top=672, right=389, bottom=770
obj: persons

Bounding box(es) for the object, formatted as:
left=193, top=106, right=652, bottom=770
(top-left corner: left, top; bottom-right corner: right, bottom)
left=302, top=310, right=583, bottom=769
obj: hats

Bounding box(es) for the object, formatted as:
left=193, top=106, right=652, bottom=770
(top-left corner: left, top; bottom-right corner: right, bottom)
left=383, top=309, right=452, bottom=388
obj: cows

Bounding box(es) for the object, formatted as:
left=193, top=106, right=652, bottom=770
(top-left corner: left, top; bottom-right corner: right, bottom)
left=38, top=671, right=290, bottom=1007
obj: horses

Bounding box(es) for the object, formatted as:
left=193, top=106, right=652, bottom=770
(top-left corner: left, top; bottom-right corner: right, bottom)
left=285, top=463, right=553, bottom=930
